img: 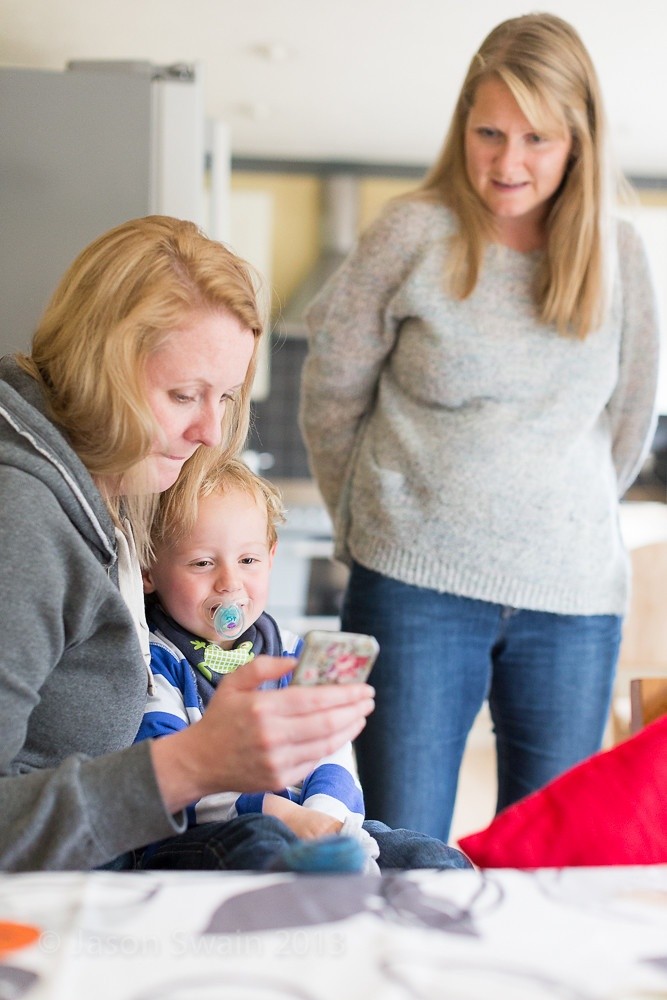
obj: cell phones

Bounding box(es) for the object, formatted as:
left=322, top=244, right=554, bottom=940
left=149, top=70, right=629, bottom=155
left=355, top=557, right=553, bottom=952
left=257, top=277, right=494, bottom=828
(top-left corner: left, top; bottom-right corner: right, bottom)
left=288, top=628, right=379, bottom=684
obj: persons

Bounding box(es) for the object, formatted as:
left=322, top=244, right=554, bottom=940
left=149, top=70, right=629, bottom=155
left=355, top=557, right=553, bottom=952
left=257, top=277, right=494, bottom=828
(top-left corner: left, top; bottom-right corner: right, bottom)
left=0, top=212, right=263, bottom=873
left=123, top=451, right=479, bottom=872
left=293, top=11, right=664, bottom=846
